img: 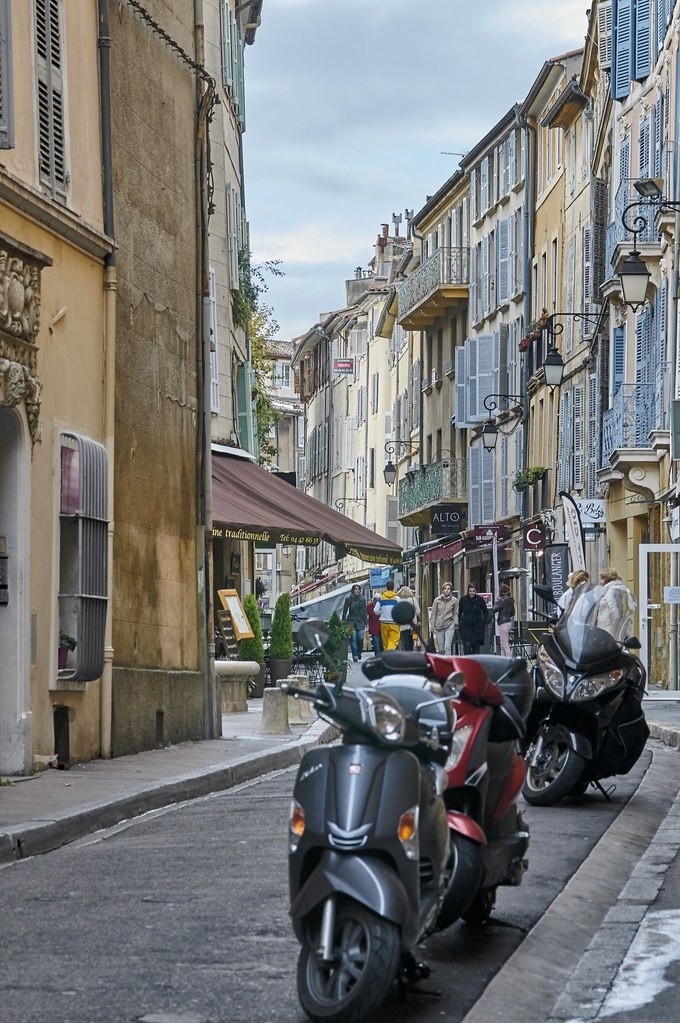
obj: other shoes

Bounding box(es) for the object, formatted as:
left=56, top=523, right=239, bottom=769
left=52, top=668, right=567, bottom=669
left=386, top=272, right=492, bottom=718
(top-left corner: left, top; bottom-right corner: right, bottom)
left=354, top=658, right=357, bottom=662
left=358, top=653, right=361, bottom=660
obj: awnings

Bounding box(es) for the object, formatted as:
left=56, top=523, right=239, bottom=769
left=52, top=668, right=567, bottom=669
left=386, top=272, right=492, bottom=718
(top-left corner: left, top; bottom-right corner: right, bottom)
left=291, top=573, right=339, bottom=598
left=210, top=451, right=403, bottom=564
left=422, top=518, right=543, bottom=567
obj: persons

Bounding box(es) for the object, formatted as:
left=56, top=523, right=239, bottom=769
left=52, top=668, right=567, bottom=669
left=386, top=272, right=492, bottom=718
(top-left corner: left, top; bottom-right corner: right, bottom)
left=459, top=583, right=489, bottom=655
left=430, top=582, right=459, bottom=655
left=492, top=585, right=515, bottom=657
left=367, top=596, right=383, bottom=655
left=397, top=586, right=421, bottom=651
left=373, top=581, right=402, bottom=651
left=600, top=568, right=635, bottom=644
left=557, top=571, right=598, bottom=626
left=342, top=584, right=368, bottom=662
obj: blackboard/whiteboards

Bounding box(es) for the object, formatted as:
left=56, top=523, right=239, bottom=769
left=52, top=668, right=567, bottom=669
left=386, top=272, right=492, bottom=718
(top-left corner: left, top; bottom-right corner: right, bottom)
left=217, top=589, right=255, bottom=641
left=217, top=610, right=240, bottom=661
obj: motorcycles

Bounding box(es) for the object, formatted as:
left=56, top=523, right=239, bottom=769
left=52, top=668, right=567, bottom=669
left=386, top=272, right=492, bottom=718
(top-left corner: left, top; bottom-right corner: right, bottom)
left=271, top=577, right=653, bottom=1023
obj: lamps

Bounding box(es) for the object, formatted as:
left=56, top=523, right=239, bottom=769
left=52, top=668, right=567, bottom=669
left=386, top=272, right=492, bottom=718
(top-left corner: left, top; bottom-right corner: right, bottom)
left=614, top=198, right=680, bottom=312
left=482, top=394, right=524, bottom=456
left=542, top=310, right=608, bottom=390
left=383, top=440, right=420, bottom=487
left=280, top=543, right=292, bottom=558
left=634, top=175, right=664, bottom=196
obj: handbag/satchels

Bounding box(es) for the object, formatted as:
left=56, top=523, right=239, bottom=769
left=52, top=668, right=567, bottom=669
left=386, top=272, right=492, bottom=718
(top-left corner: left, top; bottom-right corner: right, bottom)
left=426, top=631, right=436, bottom=653
left=411, top=623, right=419, bottom=640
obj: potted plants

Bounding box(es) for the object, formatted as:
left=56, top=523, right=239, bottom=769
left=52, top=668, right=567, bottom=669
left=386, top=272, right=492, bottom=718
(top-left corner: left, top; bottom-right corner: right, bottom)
left=241, top=593, right=266, bottom=698
left=325, top=609, right=348, bottom=682
left=268, top=592, right=295, bottom=686
left=517, top=308, right=550, bottom=352
